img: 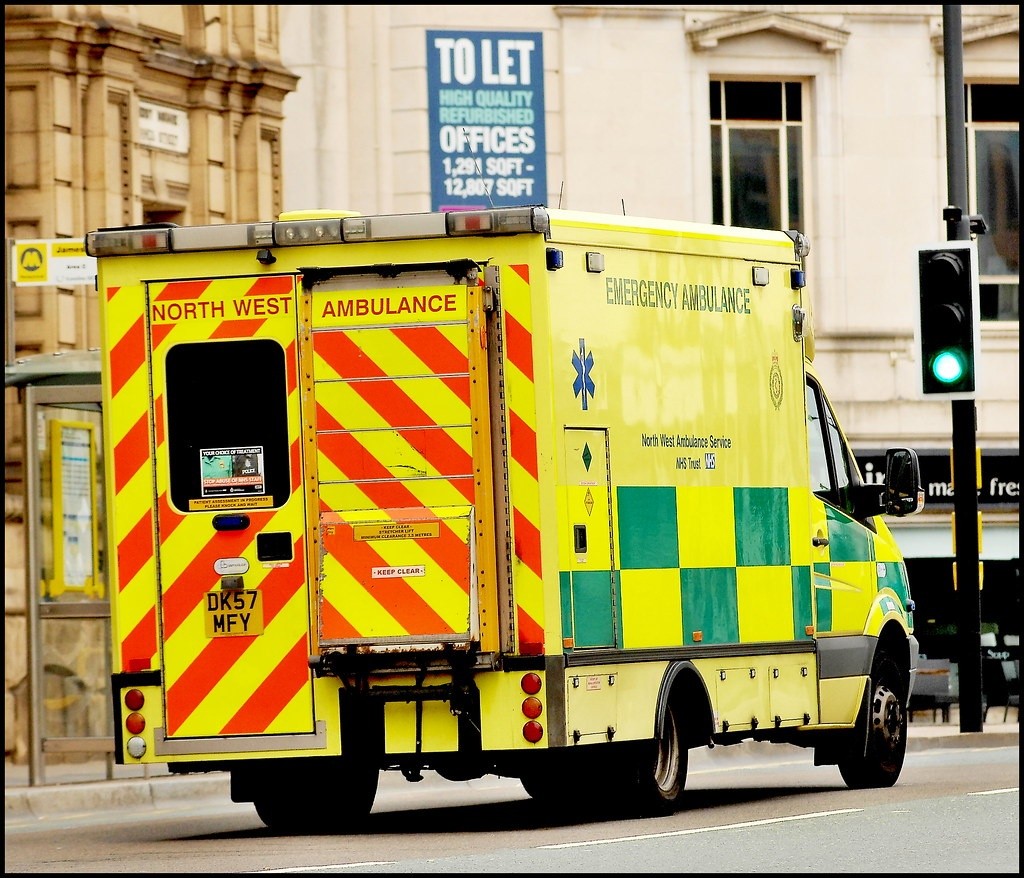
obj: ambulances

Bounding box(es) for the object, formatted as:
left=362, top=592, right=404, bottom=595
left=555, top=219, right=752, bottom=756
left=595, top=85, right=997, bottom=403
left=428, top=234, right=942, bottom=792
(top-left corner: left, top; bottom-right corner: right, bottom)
left=85, top=198, right=926, bottom=838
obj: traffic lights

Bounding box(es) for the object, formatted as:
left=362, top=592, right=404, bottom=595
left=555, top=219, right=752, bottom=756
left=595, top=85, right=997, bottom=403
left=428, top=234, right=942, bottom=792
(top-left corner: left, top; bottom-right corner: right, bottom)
left=915, top=240, right=981, bottom=404
